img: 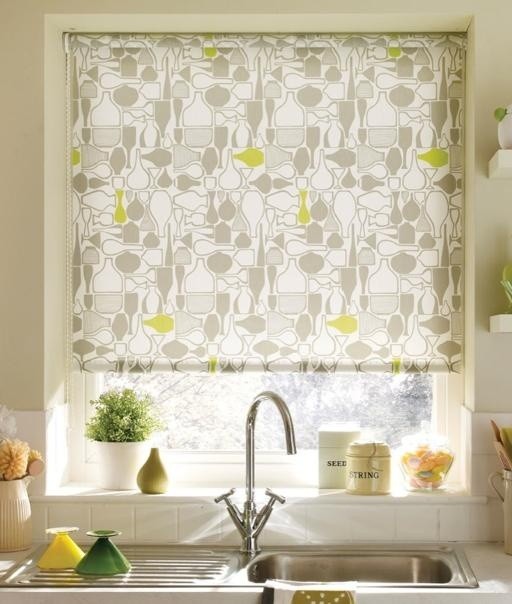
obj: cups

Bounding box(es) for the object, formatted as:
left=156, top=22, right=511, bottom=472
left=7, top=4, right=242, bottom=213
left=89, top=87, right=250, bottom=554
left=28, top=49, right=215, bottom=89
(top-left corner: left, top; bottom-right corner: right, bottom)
left=488, top=467, right=512, bottom=556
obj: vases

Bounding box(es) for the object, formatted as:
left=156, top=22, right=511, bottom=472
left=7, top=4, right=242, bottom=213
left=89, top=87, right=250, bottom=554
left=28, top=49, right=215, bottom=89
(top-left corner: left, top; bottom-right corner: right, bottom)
left=134, top=447, right=170, bottom=494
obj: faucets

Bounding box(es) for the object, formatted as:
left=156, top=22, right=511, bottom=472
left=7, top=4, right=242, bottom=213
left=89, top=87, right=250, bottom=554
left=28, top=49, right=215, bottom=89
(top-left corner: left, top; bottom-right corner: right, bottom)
left=246, top=391, right=297, bottom=502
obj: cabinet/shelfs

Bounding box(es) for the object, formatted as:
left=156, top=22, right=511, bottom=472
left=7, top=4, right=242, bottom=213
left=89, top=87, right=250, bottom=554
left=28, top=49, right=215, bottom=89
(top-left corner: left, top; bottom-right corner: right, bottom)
left=486, top=147, right=512, bottom=334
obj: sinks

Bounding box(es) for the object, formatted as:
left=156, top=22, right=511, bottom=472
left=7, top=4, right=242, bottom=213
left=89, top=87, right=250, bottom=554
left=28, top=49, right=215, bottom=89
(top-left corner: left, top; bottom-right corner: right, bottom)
left=243, top=544, right=478, bottom=588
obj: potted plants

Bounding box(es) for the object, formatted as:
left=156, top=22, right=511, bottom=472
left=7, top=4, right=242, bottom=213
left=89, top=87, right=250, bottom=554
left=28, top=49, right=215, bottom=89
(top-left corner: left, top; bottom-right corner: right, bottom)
left=84, top=386, right=161, bottom=491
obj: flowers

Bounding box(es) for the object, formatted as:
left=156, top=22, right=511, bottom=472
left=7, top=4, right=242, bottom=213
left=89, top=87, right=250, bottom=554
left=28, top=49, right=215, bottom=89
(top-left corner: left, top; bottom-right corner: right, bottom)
left=0, top=404, right=45, bottom=480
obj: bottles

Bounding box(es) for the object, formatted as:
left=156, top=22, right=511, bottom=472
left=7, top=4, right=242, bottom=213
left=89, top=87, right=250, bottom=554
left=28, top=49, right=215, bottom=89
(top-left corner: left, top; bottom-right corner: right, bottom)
left=343, top=440, right=394, bottom=497
left=317, top=429, right=362, bottom=489
left=396, top=432, right=458, bottom=493
